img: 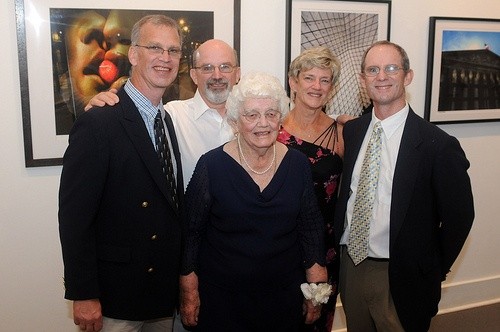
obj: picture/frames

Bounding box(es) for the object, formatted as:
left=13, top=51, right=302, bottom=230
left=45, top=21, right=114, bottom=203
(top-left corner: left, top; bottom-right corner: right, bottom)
left=423, top=16, right=500, bottom=124
left=284, top=0, right=393, bottom=121
left=14, top=0, right=242, bottom=168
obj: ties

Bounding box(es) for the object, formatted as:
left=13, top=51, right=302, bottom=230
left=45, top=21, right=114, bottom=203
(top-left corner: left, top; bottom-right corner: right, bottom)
left=348, top=122, right=383, bottom=265
left=155, top=110, right=177, bottom=206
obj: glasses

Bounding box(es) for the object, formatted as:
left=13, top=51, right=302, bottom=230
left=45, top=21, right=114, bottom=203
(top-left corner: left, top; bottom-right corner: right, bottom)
left=136, top=45, right=182, bottom=56
left=238, top=110, right=282, bottom=122
left=363, top=65, right=408, bottom=77
left=193, top=64, right=238, bottom=73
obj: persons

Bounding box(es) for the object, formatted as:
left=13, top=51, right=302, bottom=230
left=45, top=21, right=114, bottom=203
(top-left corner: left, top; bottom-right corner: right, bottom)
left=333, top=40, right=475, bottom=332
left=181, top=71, right=332, bottom=332
left=58, top=14, right=183, bottom=332
left=65, top=9, right=165, bottom=106
left=282, top=48, right=358, bottom=332
left=86, top=39, right=355, bottom=332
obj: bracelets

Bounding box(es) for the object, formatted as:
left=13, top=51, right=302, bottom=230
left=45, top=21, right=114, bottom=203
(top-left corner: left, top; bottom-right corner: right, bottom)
left=300, top=280, right=333, bottom=307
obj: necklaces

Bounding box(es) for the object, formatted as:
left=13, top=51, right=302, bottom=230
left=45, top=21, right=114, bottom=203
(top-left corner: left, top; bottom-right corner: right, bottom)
left=238, top=135, right=275, bottom=174
